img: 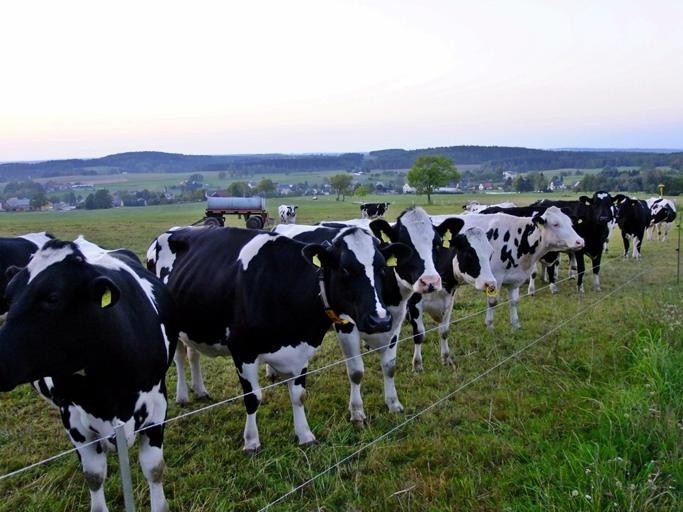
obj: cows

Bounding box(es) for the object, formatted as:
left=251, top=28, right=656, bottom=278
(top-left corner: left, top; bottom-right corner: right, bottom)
left=0, top=230, right=56, bottom=313
left=0, top=233, right=180, bottom=512
left=144, top=223, right=415, bottom=457
left=263, top=191, right=676, bottom=430
